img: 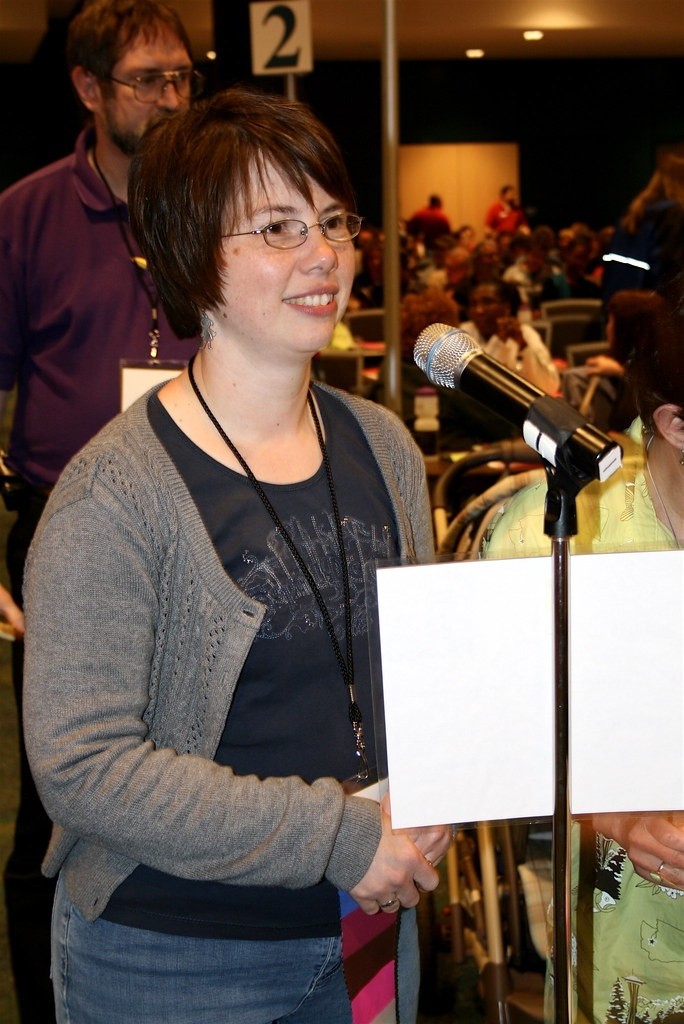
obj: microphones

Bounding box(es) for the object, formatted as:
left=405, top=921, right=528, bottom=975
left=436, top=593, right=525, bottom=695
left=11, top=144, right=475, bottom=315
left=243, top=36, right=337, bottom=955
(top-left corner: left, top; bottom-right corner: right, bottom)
left=413, top=322, right=623, bottom=482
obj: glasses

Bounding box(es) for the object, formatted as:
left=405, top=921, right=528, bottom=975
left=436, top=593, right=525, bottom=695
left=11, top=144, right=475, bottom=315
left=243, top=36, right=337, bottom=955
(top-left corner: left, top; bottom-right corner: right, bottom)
left=87, top=65, right=206, bottom=105
left=215, top=212, right=367, bottom=250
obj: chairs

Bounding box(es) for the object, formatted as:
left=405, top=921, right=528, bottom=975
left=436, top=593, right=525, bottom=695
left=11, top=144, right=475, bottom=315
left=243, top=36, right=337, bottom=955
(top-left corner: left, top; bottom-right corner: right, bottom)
left=314, top=299, right=625, bottom=1024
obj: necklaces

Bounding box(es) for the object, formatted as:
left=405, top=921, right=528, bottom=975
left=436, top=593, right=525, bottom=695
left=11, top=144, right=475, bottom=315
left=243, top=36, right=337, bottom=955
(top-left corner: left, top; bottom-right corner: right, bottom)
left=646, top=434, right=680, bottom=550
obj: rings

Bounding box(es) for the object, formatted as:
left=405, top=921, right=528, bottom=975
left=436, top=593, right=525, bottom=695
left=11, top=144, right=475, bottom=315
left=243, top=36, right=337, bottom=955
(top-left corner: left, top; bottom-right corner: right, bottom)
left=380, top=898, right=397, bottom=907
left=649, top=862, right=664, bottom=884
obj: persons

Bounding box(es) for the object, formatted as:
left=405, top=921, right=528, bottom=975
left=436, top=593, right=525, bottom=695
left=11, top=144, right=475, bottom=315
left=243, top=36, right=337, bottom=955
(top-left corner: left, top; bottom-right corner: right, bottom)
left=476, top=152, right=684, bottom=1024
left=345, top=184, right=616, bottom=392
left=0, top=0, right=235, bottom=1024
left=23, top=90, right=454, bottom=1024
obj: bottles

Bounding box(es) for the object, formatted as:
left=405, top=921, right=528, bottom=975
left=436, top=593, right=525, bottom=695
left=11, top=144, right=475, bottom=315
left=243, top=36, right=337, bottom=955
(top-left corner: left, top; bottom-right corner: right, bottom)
left=412, top=387, right=441, bottom=465
